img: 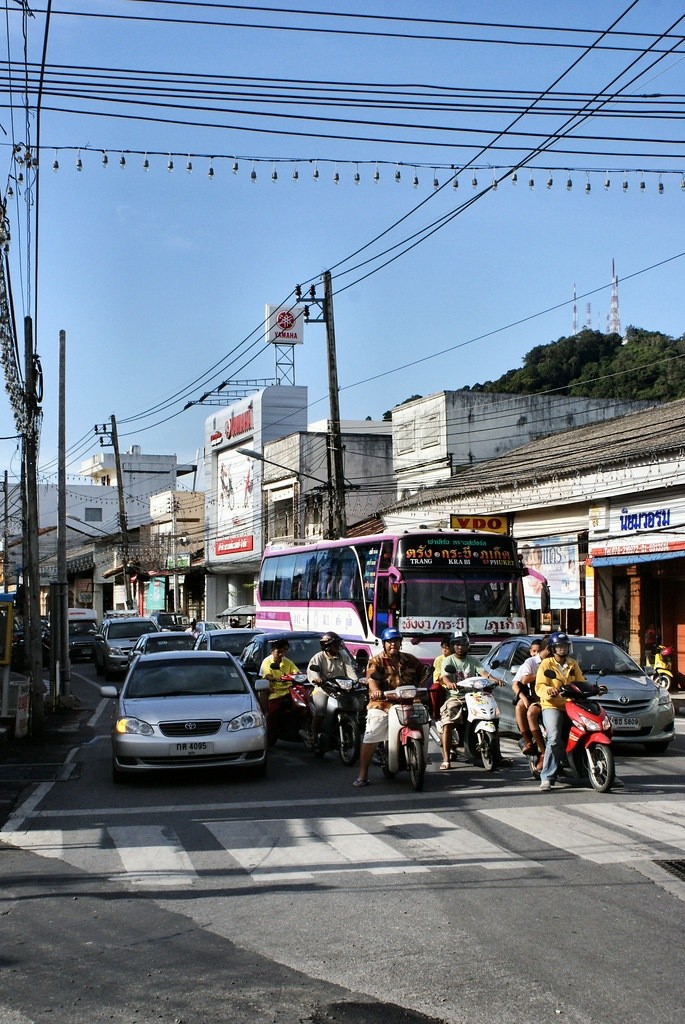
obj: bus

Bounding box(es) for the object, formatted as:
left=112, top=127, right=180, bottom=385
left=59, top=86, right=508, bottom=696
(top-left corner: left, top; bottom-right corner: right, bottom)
left=253, top=527, right=550, bottom=673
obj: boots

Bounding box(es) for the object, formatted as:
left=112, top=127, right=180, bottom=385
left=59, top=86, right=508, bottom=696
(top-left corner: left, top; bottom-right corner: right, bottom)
left=532, top=728, right=546, bottom=769
left=522, top=730, right=538, bottom=756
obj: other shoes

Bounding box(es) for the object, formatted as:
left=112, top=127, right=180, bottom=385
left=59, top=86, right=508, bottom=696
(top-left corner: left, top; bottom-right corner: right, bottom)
left=540, top=780, right=551, bottom=791
left=613, top=777, right=624, bottom=787
left=439, top=762, right=451, bottom=769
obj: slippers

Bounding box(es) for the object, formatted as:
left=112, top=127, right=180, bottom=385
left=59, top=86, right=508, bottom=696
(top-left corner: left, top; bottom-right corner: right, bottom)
left=352, top=778, right=370, bottom=787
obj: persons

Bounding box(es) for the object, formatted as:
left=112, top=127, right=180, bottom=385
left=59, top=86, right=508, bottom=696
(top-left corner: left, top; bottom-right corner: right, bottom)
left=259, top=638, right=302, bottom=736
left=512, top=632, right=607, bottom=790
left=307, top=632, right=363, bottom=736
left=429, top=631, right=504, bottom=770
left=521, top=544, right=542, bottom=597
left=352, top=627, right=432, bottom=786
left=184, top=617, right=203, bottom=648
left=645, top=624, right=658, bottom=667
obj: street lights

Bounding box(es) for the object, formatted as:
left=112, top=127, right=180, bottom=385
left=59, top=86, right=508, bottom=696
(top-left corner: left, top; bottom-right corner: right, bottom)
left=237, top=448, right=348, bottom=538
left=66, top=514, right=133, bottom=610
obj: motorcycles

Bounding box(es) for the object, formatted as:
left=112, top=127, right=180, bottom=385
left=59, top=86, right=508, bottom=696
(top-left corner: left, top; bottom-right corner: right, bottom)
left=645, top=643, right=674, bottom=691
left=359, top=665, right=435, bottom=794
left=268, top=663, right=369, bottom=766
left=517, top=668, right=616, bottom=793
left=428, top=660, right=506, bottom=772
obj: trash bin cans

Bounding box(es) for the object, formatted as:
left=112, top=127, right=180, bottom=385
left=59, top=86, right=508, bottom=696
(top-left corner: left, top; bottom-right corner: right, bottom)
left=116, top=603, right=125, bottom=611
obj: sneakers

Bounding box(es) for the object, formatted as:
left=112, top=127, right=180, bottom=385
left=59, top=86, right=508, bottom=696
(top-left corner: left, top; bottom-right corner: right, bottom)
left=304, top=736, right=321, bottom=753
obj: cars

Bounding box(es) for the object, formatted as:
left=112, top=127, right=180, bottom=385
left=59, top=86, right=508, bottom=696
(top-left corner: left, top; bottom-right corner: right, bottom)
left=0, top=608, right=234, bottom=670
left=192, top=629, right=267, bottom=658
left=236, top=631, right=368, bottom=681
left=99, top=650, right=269, bottom=785
left=127, top=631, right=197, bottom=671
left=476, top=635, right=676, bottom=754
left=93, top=617, right=170, bottom=682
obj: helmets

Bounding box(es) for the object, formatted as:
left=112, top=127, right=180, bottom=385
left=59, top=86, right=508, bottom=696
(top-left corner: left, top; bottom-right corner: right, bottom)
left=450, top=631, right=470, bottom=652
left=661, top=648, right=673, bottom=662
left=320, top=632, right=343, bottom=652
left=381, top=627, right=403, bottom=640
left=549, top=631, right=570, bottom=657
